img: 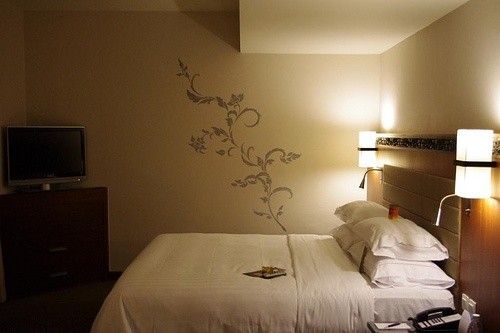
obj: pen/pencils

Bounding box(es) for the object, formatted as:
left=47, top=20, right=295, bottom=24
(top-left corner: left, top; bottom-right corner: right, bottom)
left=388, top=323, right=400, bottom=327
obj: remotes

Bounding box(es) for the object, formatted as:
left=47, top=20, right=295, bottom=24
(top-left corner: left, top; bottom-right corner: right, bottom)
left=263, top=272, right=286, bottom=278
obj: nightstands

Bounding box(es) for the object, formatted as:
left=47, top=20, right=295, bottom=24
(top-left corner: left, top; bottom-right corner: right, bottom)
left=367, top=321, right=417, bottom=333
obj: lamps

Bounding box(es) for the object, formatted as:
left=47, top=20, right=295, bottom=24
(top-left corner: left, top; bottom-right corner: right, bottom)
left=435, top=194, right=472, bottom=227
left=358, top=131, right=383, bottom=167
left=428, top=129, right=494, bottom=199
left=359, top=167, right=384, bottom=189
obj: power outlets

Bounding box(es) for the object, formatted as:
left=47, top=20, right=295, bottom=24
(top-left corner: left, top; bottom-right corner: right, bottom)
left=462, top=293, right=476, bottom=316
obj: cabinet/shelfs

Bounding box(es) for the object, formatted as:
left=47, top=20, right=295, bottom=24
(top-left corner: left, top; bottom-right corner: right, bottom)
left=0, top=186, right=109, bottom=302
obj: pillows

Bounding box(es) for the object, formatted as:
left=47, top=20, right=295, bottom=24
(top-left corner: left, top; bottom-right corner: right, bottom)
left=329, top=201, right=456, bottom=289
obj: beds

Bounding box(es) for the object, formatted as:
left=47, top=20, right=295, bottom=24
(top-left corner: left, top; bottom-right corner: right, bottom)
left=90, top=165, right=463, bottom=333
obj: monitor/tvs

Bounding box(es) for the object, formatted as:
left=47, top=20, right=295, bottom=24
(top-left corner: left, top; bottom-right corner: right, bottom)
left=6, top=126, right=87, bottom=186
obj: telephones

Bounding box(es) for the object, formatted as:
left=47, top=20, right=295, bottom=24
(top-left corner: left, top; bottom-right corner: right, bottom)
left=414, top=307, right=462, bottom=333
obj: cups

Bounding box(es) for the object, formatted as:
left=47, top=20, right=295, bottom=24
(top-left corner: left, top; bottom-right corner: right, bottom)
left=389, top=203, right=400, bottom=220
